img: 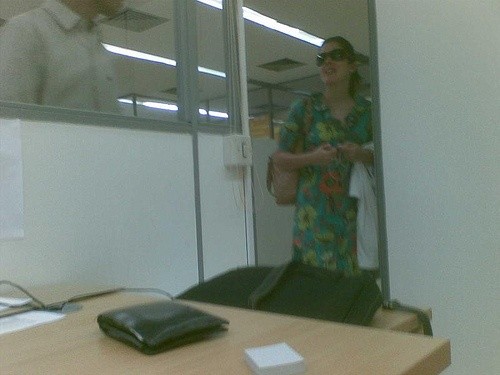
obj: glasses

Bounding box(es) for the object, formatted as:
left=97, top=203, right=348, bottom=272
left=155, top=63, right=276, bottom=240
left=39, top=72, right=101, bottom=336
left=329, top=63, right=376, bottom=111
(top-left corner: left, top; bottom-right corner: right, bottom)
left=317, top=50, right=353, bottom=66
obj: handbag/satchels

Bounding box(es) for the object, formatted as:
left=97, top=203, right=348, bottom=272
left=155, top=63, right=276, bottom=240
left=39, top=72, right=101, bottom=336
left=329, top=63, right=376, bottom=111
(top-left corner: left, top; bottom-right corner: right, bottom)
left=267, top=96, right=313, bottom=206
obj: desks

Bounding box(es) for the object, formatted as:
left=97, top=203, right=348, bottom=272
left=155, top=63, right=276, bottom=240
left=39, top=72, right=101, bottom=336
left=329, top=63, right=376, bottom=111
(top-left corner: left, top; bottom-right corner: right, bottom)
left=1, top=286, right=453, bottom=375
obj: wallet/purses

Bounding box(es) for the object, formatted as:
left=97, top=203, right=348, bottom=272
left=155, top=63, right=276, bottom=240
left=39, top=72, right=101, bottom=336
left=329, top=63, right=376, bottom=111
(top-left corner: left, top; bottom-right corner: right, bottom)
left=99, top=301, right=227, bottom=356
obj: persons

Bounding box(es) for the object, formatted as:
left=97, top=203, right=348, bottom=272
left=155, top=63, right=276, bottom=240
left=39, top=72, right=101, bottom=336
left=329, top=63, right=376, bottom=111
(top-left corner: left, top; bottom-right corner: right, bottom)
left=272, top=36, right=377, bottom=276
left=1, top=1, right=124, bottom=116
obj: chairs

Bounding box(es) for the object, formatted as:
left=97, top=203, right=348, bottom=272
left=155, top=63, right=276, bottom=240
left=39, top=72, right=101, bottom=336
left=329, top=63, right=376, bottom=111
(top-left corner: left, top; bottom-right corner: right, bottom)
left=175, top=264, right=434, bottom=334
